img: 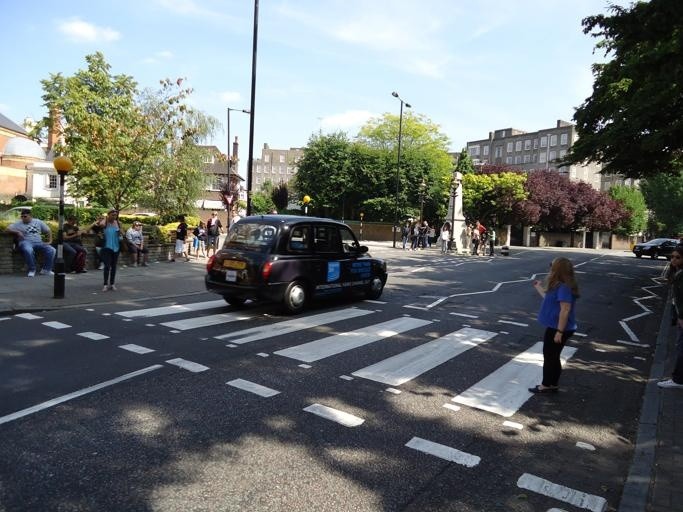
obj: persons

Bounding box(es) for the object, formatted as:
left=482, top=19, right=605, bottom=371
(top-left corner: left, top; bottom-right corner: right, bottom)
left=61, top=215, right=88, bottom=275
left=529, top=258, right=577, bottom=394
left=656, top=245, right=683, bottom=390
left=86, top=211, right=225, bottom=270
left=92, top=211, right=123, bottom=292
left=3, top=209, right=57, bottom=278
left=402, top=218, right=497, bottom=256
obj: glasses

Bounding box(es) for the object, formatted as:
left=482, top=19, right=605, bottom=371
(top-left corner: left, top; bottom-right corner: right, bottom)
left=669, top=255, right=681, bottom=260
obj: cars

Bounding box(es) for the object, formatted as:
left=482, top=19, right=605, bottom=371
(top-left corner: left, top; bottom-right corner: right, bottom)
left=632, top=237, right=679, bottom=260
left=203, top=213, right=388, bottom=316
left=1, top=206, right=34, bottom=214
left=130, top=212, right=157, bottom=217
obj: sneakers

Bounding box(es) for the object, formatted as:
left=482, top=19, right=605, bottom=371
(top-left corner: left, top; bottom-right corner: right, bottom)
left=39, top=267, right=56, bottom=276
left=28, top=268, right=38, bottom=277
left=657, top=377, right=683, bottom=390
left=97, top=262, right=105, bottom=270
left=102, top=285, right=110, bottom=292
left=111, top=284, right=119, bottom=291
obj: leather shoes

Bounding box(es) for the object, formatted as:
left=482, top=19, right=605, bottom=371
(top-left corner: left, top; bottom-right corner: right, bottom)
left=528, top=384, right=558, bottom=395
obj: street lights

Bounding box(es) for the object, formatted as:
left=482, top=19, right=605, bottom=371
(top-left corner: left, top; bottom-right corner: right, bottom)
left=391, top=90, right=413, bottom=250
left=224, top=106, right=250, bottom=236
left=445, top=176, right=461, bottom=252
left=302, top=194, right=310, bottom=215
left=417, top=176, right=428, bottom=225
left=52, top=154, right=75, bottom=301
